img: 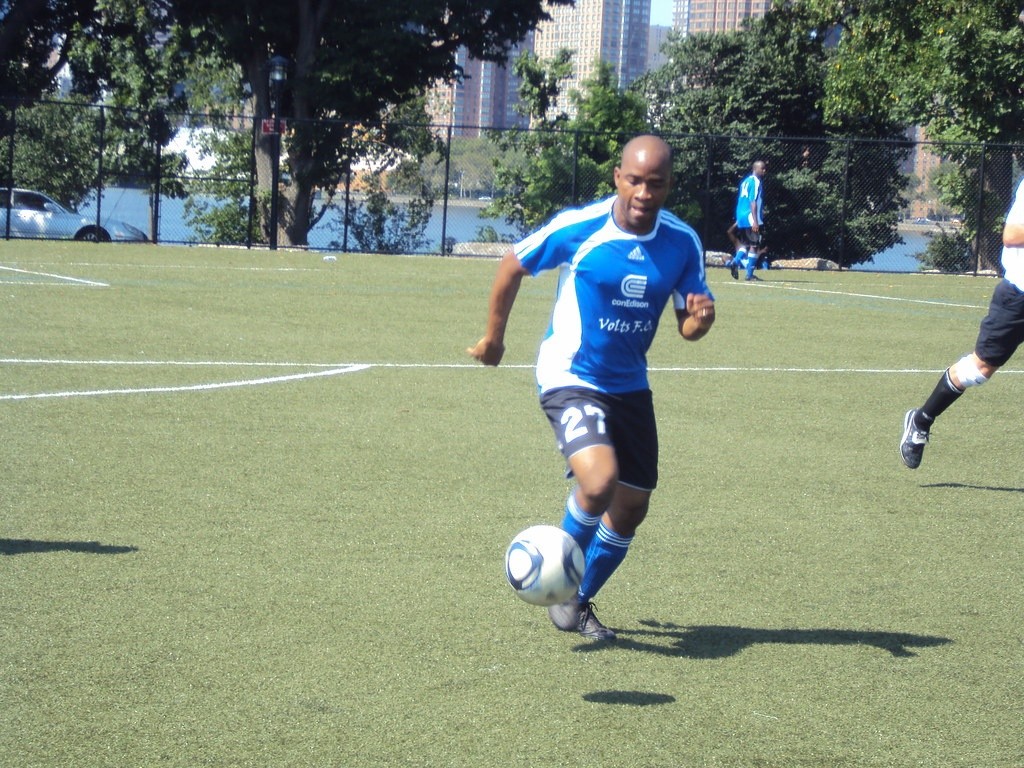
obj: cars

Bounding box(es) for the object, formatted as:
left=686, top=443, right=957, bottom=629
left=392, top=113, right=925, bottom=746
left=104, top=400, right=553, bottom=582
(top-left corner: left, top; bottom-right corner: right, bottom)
left=0, top=186, right=150, bottom=244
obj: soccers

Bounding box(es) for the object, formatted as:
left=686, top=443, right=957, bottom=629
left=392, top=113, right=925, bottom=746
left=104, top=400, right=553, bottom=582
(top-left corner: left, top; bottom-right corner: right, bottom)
left=504, top=525, right=585, bottom=607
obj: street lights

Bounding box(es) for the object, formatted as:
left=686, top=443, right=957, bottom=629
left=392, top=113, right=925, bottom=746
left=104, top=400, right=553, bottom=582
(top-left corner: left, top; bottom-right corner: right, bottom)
left=269, top=56, right=288, bottom=249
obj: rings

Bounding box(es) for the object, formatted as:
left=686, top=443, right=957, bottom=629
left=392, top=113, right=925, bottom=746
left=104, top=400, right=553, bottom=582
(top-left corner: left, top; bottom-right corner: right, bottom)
left=703, top=307, right=706, bottom=316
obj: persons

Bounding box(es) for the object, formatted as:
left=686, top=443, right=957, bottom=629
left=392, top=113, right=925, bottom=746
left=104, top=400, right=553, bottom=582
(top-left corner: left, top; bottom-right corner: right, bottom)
left=722, top=221, right=772, bottom=270
left=900, top=177, right=1024, bottom=466
left=465, top=134, right=716, bottom=641
left=731, top=160, right=766, bottom=281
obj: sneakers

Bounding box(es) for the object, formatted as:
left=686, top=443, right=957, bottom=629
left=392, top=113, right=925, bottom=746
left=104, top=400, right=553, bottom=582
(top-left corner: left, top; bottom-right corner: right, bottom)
left=899, top=409, right=933, bottom=469
left=548, top=593, right=580, bottom=631
left=745, top=274, right=763, bottom=281
left=730, top=262, right=739, bottom=279
left=575, top=601, right=616, bottom=640
